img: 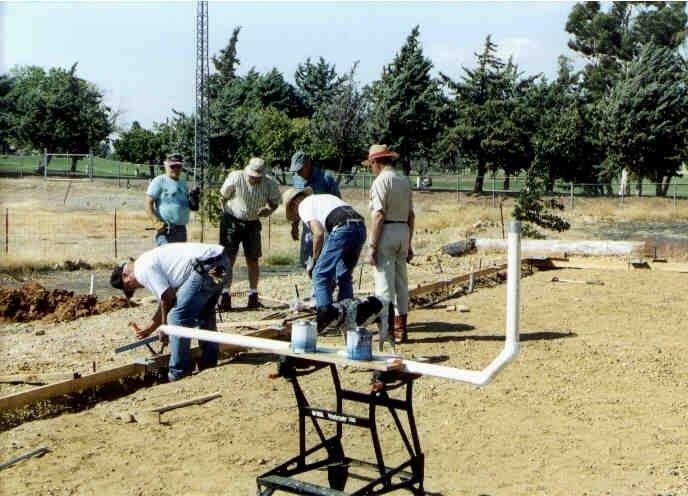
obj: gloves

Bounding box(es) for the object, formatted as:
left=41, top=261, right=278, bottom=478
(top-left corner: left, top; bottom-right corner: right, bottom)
left=257, top=201, right=274, bottom=216
left=292, top=223, right=300, bottom=241
left=154, top=220, right=165, bottom=231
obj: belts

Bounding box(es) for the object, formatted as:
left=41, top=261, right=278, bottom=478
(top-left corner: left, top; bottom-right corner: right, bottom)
left=197, top=250, right=226, bottom=266
left=347, top=221, right=364, bottom=226
left=383, top=220, right=410, bottom=224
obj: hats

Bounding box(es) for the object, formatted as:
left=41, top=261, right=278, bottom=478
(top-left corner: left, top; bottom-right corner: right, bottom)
left=244, top=156, right=268, bottom=177
left=289, top=151, right=309, bottom=171
left=281, top=186, right=313, bottom=222
left=166, top=152, right=184, bottom=164
left=361, top=145, right=400, bottom=166
left=110, top=262, right=136, bottom=300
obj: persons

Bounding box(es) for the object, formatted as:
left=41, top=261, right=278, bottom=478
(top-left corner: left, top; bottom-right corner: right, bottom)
left=290, top=151, right=341, bottom=268
left=219, top=157, right=283, bottom=310
left=362, top=144, right=415, bottom=346
left=281, top=188, right=367, bottom=308
left=110, top=241, right=232, bottom=382
left=145, top=154, right=190, bottom=243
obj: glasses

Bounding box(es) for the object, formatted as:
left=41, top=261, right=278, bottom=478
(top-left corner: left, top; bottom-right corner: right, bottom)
left=169, top=165, right=181, bottom=169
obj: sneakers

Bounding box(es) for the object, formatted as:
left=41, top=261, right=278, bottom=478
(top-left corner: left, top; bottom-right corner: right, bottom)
left=215, top=294, right=232, bottom=311
left=395, top=328, right=408, bottom=341
left=315, top=305, right=338, bottom=334
left=248, top=294, right=264, bottom=309
